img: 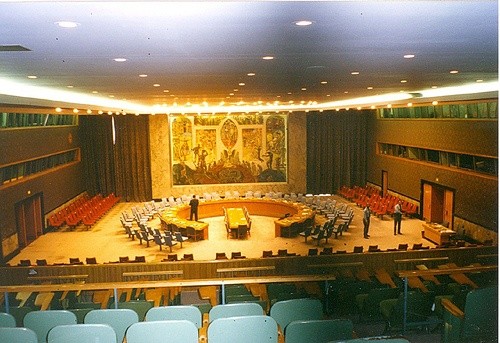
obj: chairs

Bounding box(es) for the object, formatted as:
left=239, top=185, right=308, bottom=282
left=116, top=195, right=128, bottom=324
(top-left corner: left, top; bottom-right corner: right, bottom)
left=0, top=182, right=495, bottom=266
left=0, top=280, right=498, bottom=343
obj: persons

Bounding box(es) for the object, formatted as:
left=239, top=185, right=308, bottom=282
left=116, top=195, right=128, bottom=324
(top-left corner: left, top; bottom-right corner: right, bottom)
left=189, top=195, right=199, bottom=221
left=363, top=203, right=370, bottom=239
left=394, top=201, right=404, bottom=235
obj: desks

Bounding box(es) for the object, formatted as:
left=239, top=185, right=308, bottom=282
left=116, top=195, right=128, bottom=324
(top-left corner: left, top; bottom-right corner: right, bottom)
left=269, top=198, right=285, bottom=217
left=161, top=210, right=178, bottom=217
left=172, top=205, right=189, bottom=215
left=274, top=215, right=307, bottom=238
left=161, top=215, right=188, bottom=232
left=201, top=199, right=222, bottom=218
left=228, top=208, right=249, bottom=238
left=222, top=198, right=242, bottom=215
left=243, top=198, right=268, bottom=216
left=422, top=222, right=456, bottom=244
left=297, top=203, right=315, bottom=225
left=180, top=202, right=205, bottom=220
left=285, top=199, right=299, bottom=214
left=175, top=220, right=209, bottom=241
